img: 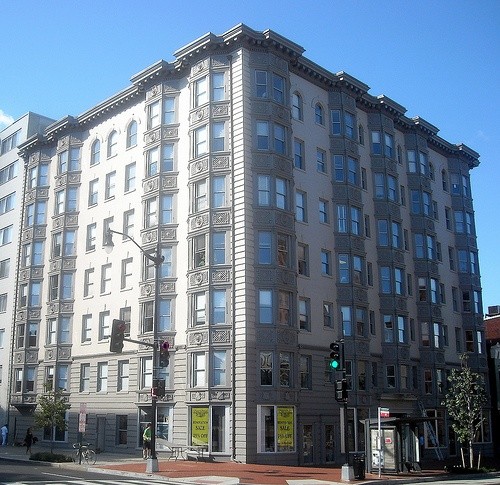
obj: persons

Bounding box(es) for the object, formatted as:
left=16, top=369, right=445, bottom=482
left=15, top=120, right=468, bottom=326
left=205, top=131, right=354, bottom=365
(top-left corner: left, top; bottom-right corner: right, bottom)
left=142, top=423, right=153, bottom=460
left=1, top=424, right=9, bottom=447
left=25, top=427, right=33, bottom=455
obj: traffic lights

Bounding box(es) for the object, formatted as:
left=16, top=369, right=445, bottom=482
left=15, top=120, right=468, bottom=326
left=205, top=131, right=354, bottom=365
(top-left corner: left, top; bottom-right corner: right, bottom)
left=160, top=339, right=170, bottom=367
left=329, top=342, right=341, bottom=370
left=110, top=319, right=125, bottom=352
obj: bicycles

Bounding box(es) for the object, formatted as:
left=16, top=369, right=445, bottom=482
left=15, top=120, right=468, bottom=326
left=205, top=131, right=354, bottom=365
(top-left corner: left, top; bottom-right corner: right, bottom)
left=71, top=443, right=97, bottom=465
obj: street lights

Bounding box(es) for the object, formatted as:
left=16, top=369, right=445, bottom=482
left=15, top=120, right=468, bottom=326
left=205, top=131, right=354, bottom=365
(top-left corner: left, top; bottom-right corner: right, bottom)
left=102, top=227, right=165, bottom=460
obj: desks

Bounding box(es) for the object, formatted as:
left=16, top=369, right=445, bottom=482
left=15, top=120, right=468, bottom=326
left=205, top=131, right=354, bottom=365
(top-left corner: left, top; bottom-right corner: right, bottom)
left=168, top=446, right=207, bottom=463
